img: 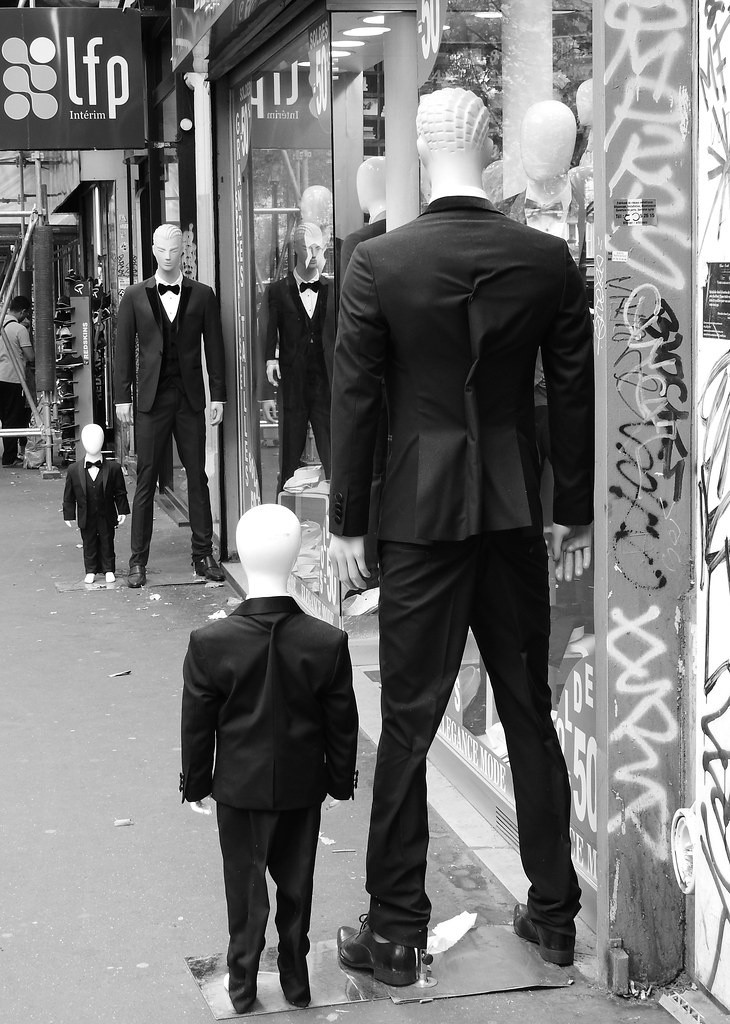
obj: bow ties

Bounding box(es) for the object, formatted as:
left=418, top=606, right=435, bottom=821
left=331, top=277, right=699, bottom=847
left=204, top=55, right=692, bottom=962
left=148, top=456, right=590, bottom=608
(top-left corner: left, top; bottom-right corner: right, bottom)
left=158, top=283, right=180, bottom=296
left=86, top=460, right=102, bottom=470
left=525, top=198, right=564, bottom=220
left=299, top=281, right=320, bottom=293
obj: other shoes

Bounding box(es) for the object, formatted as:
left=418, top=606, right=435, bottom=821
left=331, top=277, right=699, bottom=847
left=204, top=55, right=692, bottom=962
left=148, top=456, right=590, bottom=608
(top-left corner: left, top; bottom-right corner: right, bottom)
left=17, top=452, right=24, bottom=460
left=2, top=457, right=24, bottom=468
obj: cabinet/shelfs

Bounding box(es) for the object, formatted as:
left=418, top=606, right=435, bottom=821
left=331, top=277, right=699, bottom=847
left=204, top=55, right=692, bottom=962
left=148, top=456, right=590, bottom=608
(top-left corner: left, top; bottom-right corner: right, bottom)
left=52, top=280, right=108, bottom=465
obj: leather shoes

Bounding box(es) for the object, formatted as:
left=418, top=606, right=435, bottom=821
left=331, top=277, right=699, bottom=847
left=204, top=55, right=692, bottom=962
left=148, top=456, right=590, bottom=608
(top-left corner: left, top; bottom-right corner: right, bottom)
left=336, top=913, right=416, bottom=986
left=513, top=903, right=575, bottom=965
left=195, top=555, right=226, bottom=581
left=127, top=565, right=147, bottom=588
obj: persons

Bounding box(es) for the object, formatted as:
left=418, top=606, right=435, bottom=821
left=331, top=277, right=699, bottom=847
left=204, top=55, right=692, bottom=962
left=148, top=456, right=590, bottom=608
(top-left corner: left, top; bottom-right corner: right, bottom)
left=113, top=224, right=228, bottom=588
left=259, top=186, right=334, bottom=502
left=494, top=100, right=582, bottom=267
left=179, top=503, right=359, bottom=1015
left=328, top=85, right=593, bottom=985
left=576, top=78, right=593, bottom=145
left=0, top=296, right=34, bottom=467
left=340, top=156, right=387, bottom=286
left=62, top=423, right=131, bottom=585
